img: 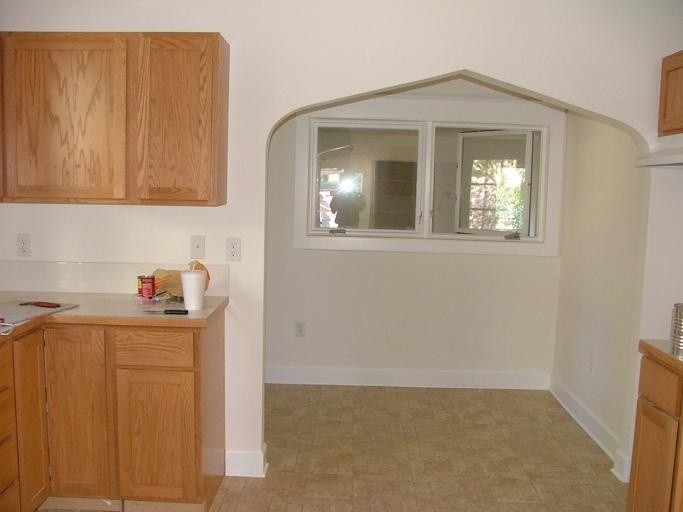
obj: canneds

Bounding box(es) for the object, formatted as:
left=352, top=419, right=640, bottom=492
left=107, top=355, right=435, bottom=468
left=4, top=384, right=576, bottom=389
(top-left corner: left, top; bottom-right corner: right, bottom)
left=137, top=275, right=155, bottom=304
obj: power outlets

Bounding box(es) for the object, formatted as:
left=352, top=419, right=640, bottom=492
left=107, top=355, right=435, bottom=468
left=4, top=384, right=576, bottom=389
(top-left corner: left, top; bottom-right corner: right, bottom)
left=16, top=234, right=29, bottom=256
left=226, top=238, right=240, bottom=260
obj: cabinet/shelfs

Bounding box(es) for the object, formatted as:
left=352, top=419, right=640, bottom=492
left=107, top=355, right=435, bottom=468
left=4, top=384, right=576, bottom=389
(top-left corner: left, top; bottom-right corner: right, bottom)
left=658, top=50, right=683, bottom=137
left=627, top=356, right=683, bottom=512
left=113, top=327, right=226, bottom=504
left=1, top=32, right=230, bottom=205
left=44, top=323, right=119, bottom=501
left=0, top=330, right=49, bottom=512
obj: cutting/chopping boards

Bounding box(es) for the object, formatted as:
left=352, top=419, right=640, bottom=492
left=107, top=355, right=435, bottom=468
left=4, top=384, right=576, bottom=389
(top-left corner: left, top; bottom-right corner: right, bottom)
left=0, top=299, right=78, bottom=328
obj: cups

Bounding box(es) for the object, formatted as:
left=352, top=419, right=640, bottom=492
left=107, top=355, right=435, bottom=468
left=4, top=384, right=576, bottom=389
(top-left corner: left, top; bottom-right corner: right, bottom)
left=180, top=269, right=207, bottom=310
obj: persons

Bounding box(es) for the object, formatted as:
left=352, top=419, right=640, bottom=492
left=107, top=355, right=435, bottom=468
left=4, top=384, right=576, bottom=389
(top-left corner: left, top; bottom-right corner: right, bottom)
left=329, top=175, right=367, bottom=228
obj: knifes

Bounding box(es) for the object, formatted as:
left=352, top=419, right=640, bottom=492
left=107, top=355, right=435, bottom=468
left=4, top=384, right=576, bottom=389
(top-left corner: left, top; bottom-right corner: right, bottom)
left=142, top=310, right=188, bottom=315
